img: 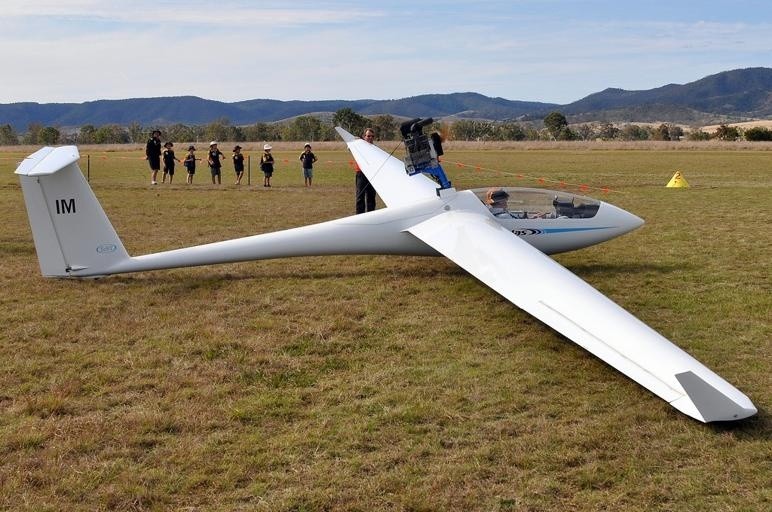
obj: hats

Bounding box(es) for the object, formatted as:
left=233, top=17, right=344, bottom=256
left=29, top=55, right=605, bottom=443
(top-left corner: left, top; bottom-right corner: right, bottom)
left=152, top=129, right=272, bottom=152
left=304, top=143, right=311, bottom=147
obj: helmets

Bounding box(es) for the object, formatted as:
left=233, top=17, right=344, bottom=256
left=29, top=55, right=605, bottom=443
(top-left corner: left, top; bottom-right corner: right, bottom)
left=486, top=188, right=510, bottom=204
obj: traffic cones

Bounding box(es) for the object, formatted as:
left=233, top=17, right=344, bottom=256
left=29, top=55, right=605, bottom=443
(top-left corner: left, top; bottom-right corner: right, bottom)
left=666, top=167, right=695, bottom=189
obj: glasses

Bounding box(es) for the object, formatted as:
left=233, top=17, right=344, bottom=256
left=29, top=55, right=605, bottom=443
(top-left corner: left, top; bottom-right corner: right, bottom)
left=367, top=135, right=375, bottom=137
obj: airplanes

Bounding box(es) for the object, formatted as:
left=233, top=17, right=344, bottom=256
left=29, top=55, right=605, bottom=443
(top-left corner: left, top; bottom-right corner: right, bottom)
left=12, top=123, right=762, bottom=429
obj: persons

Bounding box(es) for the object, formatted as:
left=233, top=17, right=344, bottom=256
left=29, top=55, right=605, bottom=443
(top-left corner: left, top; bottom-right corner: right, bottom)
left=262, top=143, right=274, bottom=187
left=347, top=128, right=376, bottom=214
left=299, top=143, right=319, bottom=187
left=144, top=129, right=245, bottom=185
left=485, top=188, right=545, bottom=219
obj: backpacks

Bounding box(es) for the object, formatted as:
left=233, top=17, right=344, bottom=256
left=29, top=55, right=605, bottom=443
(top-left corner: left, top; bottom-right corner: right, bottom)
left=183, top=159, right=189, bottom=166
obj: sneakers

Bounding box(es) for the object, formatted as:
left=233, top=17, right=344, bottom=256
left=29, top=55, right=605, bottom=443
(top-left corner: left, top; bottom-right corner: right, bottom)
left=151, top=181, right=158, bottom=185
left=235, top=181, right=240, bottom=185
left=264, top=185, right=271, bottom=187
